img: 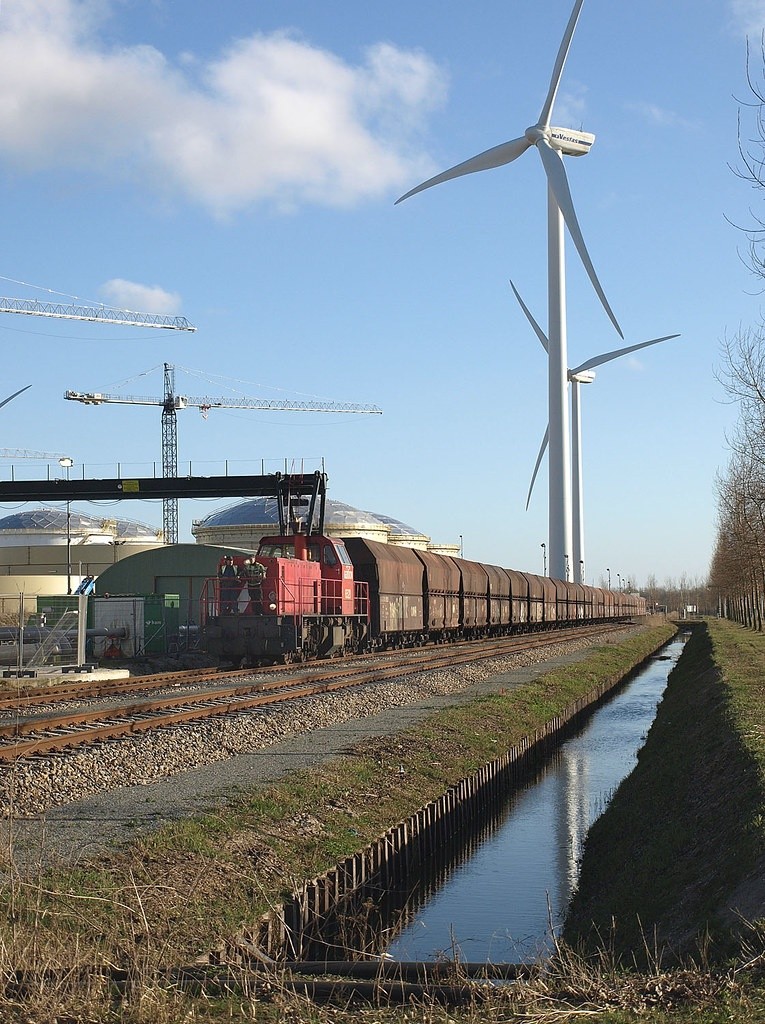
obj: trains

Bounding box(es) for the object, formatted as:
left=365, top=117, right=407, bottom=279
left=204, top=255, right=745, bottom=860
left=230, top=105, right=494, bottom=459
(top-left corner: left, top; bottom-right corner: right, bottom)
left=201, top=525, right=647, bottom=662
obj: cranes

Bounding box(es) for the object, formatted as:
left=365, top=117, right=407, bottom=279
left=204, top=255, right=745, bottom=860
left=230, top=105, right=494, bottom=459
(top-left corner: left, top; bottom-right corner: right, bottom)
left=59, top=357, right=386, bottom=544
left=0, top=274, right=200, bottom=338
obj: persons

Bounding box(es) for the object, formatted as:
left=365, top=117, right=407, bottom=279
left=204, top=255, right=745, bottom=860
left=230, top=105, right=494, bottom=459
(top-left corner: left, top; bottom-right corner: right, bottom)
left=236, top=554, right=266, bottom=615
left=218, top=556, right=243, bottom=613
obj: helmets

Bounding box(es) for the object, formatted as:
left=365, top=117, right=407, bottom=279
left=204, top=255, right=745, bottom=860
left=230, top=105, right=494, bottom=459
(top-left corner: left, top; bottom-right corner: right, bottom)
left=226, top=556, right=234, bottom=560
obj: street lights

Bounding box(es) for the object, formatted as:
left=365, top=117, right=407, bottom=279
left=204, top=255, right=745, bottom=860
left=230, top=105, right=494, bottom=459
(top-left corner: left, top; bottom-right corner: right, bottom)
left=57, top=457, right=75, bottom=594
left=541, top=543, right=631, bottom=593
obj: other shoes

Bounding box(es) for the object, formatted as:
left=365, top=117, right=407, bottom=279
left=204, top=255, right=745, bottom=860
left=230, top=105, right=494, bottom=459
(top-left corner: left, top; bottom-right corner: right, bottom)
left=233, top=612, right=239, bottom=616
left=256, top=611, right=263, bottom=616
left=220, top=612, right=228, bottom=616
left=254, top=611, right=259, bottom=616
